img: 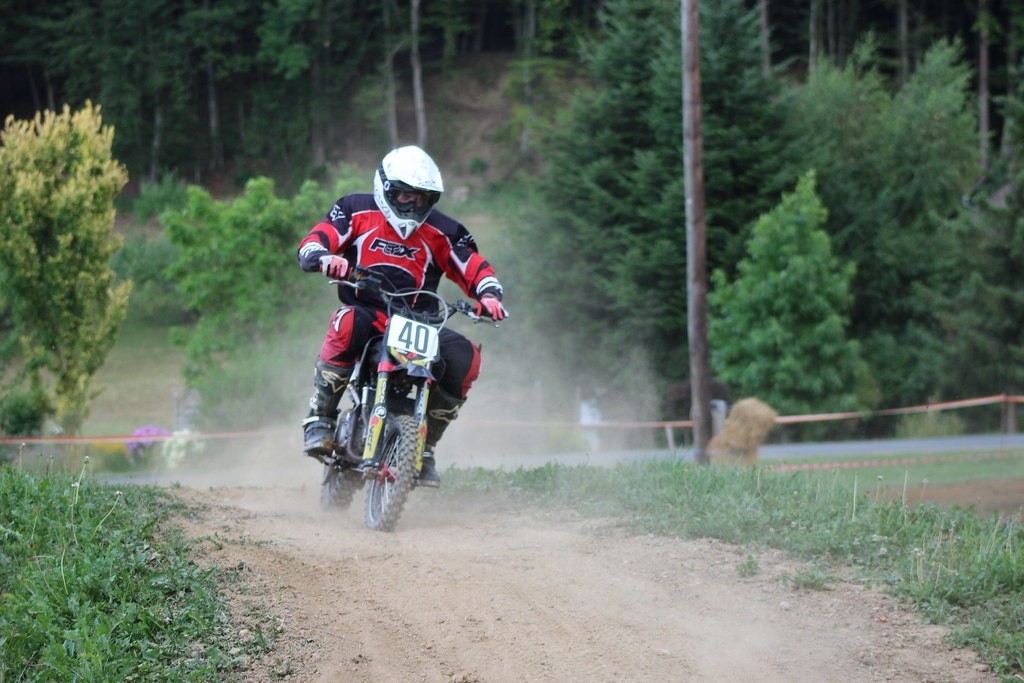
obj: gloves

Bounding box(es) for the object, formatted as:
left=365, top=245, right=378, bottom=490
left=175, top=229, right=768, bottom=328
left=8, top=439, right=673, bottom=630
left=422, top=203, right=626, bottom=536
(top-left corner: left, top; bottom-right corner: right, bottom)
left=475, top=292, right=510, bottom=321
left=318, top=254, right=349, bottom=279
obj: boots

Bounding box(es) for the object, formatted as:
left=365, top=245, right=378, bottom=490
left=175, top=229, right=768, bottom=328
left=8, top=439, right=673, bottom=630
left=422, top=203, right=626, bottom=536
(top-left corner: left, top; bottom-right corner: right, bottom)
left=303, top=354, right=356, bottom=456
left=411, top=383, right=467, bottom=488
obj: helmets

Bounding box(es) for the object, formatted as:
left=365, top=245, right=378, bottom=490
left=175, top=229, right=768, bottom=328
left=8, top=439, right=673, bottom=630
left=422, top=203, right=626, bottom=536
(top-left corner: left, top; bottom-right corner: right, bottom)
left=374, top=145, right=445, bottom=240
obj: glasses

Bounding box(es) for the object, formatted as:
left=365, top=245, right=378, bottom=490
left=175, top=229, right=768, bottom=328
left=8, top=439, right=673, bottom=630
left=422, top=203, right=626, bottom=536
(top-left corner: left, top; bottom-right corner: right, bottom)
left=385, top=182, right=435, bottom=216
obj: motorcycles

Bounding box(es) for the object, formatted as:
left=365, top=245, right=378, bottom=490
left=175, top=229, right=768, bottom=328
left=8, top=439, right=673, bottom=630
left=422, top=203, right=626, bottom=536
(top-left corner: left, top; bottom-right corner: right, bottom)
left=307, top=260, right=501, bottom=533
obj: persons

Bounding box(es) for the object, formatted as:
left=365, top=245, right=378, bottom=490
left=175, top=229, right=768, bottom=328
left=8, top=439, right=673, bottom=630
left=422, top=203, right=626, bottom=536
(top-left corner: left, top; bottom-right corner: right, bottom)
left=295, top=143, right=509, bottom=486
